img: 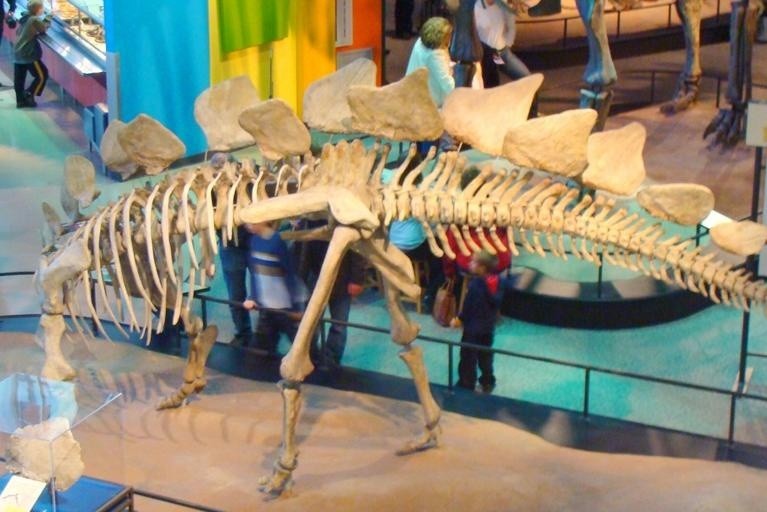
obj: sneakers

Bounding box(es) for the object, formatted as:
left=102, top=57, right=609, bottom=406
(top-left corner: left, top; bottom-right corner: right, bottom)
left=17, top=90, right=37, bottom=108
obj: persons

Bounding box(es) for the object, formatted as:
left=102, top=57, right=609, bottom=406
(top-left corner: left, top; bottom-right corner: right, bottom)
left=210, top=152, right=366, bottom=388
left=405, top=16, right=456, bottom=161
left=387, top=152, right=510, bottom=395
left=473, top=0, right=538, bottom=121
left=0, top=0, right=17, bottom=87
left=12, top=0, right=52, bottom=108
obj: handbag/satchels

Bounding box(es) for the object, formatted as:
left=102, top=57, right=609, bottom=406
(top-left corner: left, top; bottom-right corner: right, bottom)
left=433, top=278, right=457, bottom=325
